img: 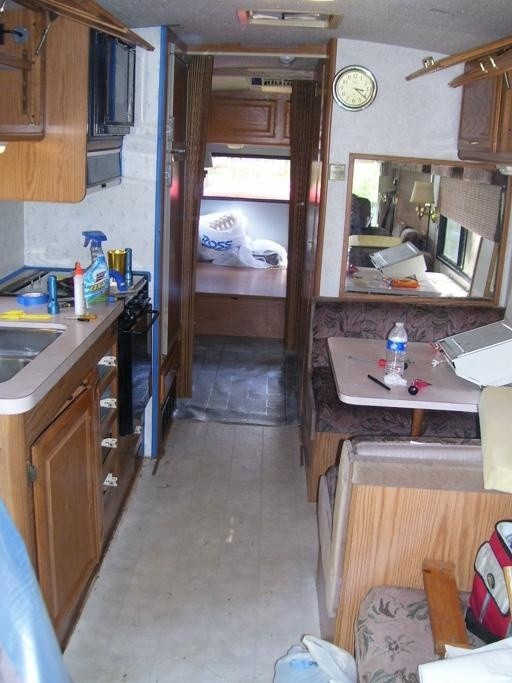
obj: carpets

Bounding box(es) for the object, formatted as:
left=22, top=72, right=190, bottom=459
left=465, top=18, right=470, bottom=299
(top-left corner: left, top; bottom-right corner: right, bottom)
left=172, top=323, right=303, bottom=428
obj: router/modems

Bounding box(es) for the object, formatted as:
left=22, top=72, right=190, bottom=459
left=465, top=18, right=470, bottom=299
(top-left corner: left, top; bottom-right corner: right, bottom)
left=16, top=291, right=49, bottom=305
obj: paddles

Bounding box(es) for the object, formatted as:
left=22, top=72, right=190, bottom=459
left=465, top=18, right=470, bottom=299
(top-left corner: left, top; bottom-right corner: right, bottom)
left=172, top=323, right=303, bottom=428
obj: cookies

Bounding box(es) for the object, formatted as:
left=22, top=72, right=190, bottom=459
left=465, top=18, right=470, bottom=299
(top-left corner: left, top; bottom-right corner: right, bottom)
left=89, top=30, right=136, bottom=140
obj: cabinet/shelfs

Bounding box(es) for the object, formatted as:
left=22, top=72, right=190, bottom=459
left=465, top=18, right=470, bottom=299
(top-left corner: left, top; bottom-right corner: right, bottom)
left=72, top=260, right=86, bottom=316
left=0, top=319, right=146, bottom=658
left=455, top=53, right=512, bottom=162
left=0, top=0, right=55, bottom=144
left=386, top=322, right=408, bottom=378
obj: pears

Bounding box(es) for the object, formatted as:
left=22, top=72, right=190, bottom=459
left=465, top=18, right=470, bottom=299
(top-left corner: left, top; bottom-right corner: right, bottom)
left=81, top=227, right=112, bottom=302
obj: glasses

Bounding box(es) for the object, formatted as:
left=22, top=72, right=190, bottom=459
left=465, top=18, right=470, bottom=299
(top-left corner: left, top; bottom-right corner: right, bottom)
left=409, top=176, right=439, bottom=226
left=379, top=171, right=401, bottom=209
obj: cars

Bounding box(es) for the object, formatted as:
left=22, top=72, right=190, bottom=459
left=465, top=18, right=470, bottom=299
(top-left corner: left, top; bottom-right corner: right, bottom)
left=108, top=249, right=127, bottom=278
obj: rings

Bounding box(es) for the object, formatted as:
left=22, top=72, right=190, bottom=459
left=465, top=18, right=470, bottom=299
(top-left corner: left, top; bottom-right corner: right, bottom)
left=63, top=315, right=98, bottom=322
left=366, top=374, right=391, bottom=392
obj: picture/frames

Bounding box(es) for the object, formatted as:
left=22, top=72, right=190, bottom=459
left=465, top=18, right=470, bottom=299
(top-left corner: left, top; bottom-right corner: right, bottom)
left=436, top=205, right=468, bottom=274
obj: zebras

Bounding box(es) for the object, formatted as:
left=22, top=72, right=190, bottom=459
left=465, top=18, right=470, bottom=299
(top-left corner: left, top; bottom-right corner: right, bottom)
left=117, top=286, right=160, bottom=436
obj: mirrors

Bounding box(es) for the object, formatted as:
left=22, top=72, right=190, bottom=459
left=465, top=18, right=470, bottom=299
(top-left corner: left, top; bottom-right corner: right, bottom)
left=339, top=150, right=511, bottom=309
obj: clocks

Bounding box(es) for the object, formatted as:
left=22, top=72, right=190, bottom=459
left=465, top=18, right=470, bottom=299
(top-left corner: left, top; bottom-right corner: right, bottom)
left=331, top=64, right=380, bottom=113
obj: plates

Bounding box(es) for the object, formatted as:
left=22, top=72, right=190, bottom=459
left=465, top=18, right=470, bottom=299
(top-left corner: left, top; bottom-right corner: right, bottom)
left=0, top=0, right=55, bottom=144
left=455, top=53, right=512, bottom=162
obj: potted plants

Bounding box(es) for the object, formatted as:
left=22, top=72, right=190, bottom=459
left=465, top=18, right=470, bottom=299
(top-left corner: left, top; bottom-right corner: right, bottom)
left=436, top=205, right=468, bottom=274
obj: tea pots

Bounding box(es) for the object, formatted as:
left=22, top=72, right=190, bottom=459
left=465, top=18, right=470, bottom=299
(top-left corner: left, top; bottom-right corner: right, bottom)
left=339, top=150, right=511, bottom=309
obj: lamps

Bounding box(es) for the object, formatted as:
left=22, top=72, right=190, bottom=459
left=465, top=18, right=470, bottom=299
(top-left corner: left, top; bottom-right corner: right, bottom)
left=379, top=171, right=401, bottom=209
left=409, top=176, right=439, bottom=226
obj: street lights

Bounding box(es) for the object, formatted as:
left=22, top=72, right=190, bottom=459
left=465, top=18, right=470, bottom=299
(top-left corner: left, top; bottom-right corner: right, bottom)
left=464, top=519, right=512, bottom=644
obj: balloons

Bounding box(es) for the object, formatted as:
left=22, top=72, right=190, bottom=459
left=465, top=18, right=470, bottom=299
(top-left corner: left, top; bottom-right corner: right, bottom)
left=331, top=64, right=380, bottom=113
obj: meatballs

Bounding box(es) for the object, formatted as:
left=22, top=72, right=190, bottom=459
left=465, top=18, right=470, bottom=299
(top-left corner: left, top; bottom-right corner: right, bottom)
left=348, top=356, right=407, bottom=370
